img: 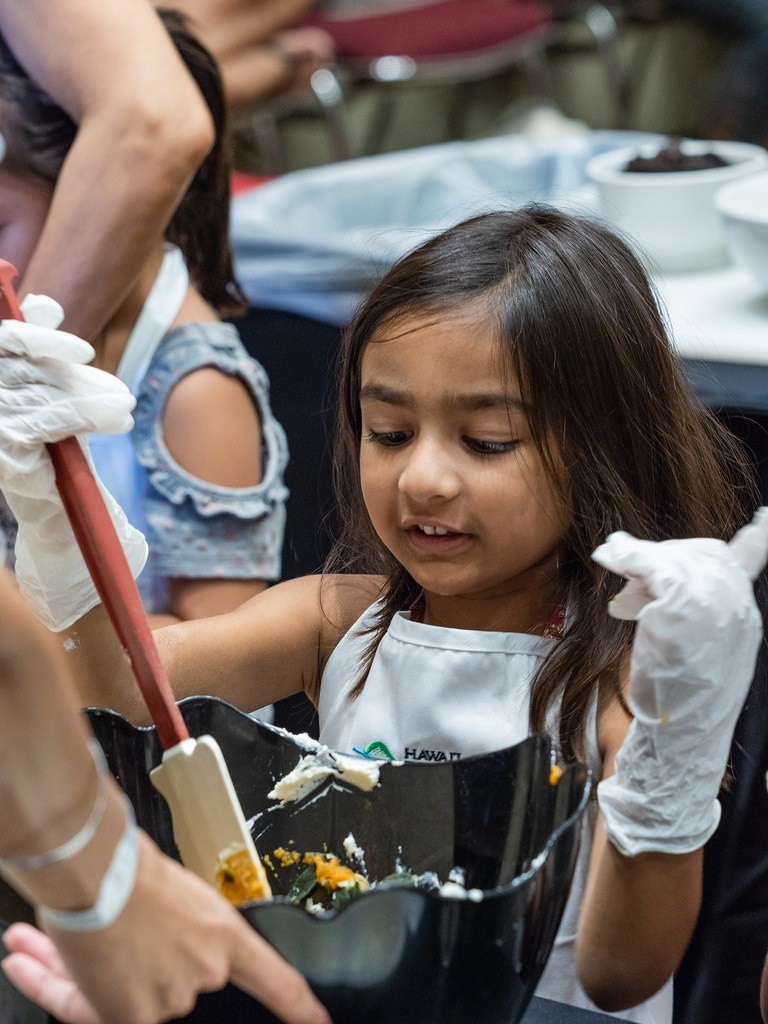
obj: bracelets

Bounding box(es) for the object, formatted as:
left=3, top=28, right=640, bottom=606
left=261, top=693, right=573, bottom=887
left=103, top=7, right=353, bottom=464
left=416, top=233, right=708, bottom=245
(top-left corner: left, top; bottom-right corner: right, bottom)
left=0, top=741, right=139, bottom=930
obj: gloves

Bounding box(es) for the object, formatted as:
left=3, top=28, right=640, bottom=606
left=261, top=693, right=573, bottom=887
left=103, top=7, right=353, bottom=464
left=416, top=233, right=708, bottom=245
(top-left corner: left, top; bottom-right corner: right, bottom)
left=0, top=292, right=149, bottom=633
left=590, top=506, right=768, bottom=857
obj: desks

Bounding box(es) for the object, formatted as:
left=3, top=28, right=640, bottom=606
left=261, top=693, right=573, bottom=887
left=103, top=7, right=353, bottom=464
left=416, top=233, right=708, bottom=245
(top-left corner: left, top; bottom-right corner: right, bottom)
left=231, top=127, right=768, bottom=411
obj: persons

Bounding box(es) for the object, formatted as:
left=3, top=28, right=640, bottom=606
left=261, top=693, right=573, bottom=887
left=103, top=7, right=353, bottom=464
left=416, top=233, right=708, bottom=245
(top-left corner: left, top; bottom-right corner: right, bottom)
left=0, top=569, right=330, bottom=1024
left=1, top=8, right=288, bottom=724
left=0, top=208, right=768, bottom=1024
left=0, top=0, right=214, bottom=340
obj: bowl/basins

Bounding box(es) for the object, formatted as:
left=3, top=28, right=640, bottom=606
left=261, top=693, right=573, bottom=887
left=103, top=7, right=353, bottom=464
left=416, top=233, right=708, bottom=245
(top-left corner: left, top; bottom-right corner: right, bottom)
left=584, top=139, right=767, bottom=273
left=714, top=174, right=768, bottom=295
left=2, top=695, right=592, bottom=1023
